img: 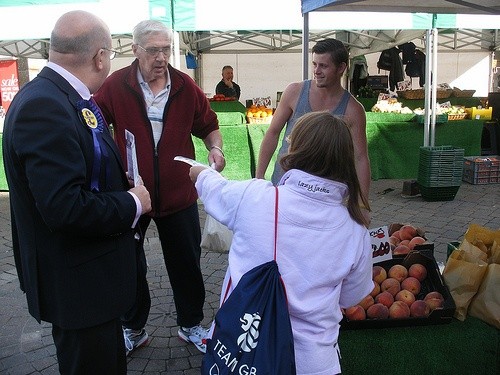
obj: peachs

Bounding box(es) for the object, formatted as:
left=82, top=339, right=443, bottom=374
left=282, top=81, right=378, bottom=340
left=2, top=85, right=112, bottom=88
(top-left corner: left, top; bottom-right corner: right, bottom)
left=345, top=263, right=445, bottom=321
left=388, top=224, right=425, bottom=255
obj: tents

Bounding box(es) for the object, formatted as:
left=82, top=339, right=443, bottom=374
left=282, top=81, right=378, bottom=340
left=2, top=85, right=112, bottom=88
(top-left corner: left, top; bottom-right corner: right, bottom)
left=299, top=0, right=500, bottom=146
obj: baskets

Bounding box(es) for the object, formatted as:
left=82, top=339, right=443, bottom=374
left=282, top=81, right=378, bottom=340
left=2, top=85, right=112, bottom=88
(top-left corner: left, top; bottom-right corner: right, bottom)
left=246, top=115, right=273, bottom=123
left=413, top=113, right=448, bottom=123
left=447, top=113, right=468, bottom=120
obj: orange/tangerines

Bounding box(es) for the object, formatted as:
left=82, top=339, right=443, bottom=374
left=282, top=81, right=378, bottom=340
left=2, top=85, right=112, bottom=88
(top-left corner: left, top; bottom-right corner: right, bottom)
left=247, top=105, right=272, bottom=119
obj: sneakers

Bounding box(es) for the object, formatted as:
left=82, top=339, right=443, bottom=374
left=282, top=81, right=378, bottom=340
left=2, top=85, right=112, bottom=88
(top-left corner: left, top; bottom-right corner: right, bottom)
left=123, top=328, right=148, bottom=357
left=178, top=325, right=210, bottom=353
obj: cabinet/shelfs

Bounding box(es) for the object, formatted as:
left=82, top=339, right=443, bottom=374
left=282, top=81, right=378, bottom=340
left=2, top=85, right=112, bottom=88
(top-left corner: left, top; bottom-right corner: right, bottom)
left=191, top=95, right=485, bottom=181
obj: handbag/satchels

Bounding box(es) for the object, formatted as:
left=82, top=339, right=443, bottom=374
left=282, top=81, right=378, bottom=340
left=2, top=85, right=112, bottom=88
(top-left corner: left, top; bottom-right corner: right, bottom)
left=201, top=259, right=297, bottom=375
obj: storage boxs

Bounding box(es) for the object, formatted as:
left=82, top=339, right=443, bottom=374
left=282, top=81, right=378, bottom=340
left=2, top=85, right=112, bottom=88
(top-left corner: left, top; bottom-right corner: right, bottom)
left=340, top=226, right=456, bottom=329
left=417, top=145, right=500, bottom=201
left=466, top=107, right=493, bottom=120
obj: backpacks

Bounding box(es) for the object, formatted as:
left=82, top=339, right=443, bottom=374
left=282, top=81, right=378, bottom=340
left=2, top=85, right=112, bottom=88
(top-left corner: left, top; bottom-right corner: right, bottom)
left=377, top=48, right=394, bottom=70
left=405, top=54, right=421, bottom=77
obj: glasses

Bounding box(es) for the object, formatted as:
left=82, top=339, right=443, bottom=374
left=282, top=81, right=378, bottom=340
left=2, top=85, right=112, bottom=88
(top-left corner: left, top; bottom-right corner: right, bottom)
left=137, top=45, right=171, bottom=55
left=92, top=47, right=116, bottom=60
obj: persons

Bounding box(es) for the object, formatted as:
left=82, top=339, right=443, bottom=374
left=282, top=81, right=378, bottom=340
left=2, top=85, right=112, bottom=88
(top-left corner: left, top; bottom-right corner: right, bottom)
left=90, top=20, right=226, bottom=356
left=216, top=66, right=240, bottom=100
left=189, top=111, right=374, bottom=375
left=3, top=12, right=153, bottom=375
left=254, top=38, right=371, bottom=228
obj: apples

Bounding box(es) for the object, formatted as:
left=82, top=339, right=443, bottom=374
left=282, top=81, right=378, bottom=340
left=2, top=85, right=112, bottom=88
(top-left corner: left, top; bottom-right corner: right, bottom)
left=208, top=94, right=236, bottom=101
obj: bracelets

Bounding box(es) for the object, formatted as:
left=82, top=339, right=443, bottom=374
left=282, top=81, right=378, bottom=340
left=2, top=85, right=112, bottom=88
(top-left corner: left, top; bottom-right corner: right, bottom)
left=358, top=203, right=368, bottom=209
left=210, top=146, right=224, bottom=157
